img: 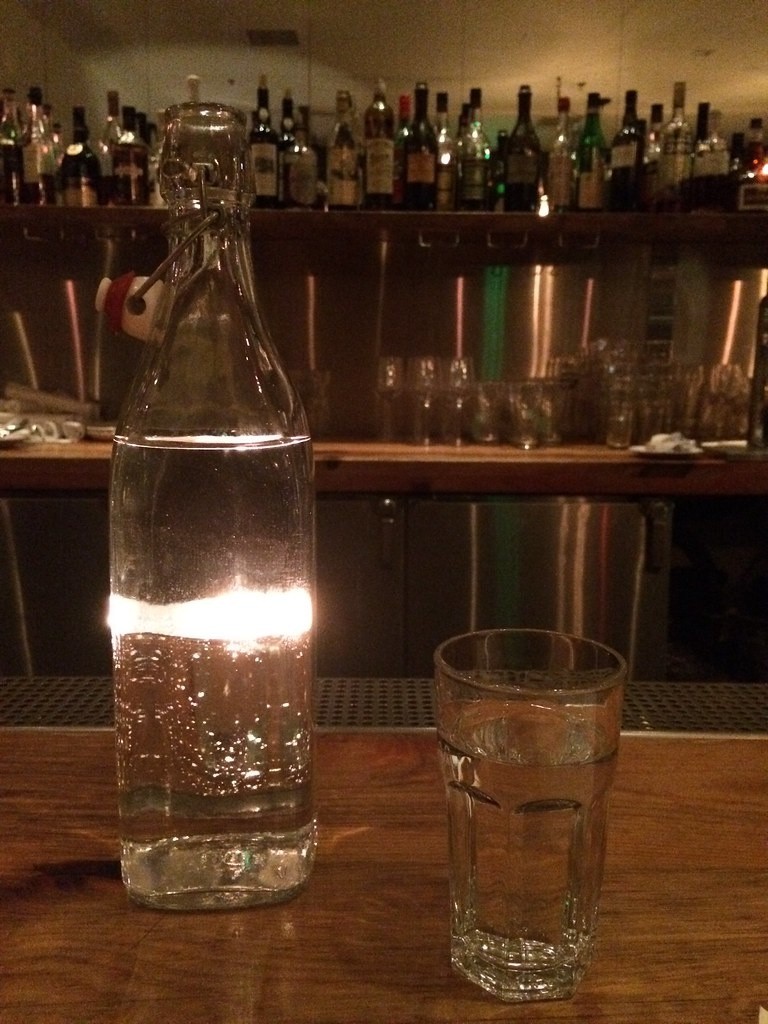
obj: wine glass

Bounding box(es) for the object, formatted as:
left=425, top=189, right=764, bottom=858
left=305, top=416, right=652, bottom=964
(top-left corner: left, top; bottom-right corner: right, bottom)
left=376, top=354, right=474, bottom=447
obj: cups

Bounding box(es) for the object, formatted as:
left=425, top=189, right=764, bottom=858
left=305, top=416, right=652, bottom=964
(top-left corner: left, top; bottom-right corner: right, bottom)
left=464, top=335, right=753, bottom=451
left=435, top=629, right=629, bottom=1005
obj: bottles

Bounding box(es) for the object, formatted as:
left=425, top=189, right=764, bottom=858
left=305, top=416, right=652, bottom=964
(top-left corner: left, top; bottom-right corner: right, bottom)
left=0, top=70, right=767, bottom=213
left=102, top=103, right=324, bottom=916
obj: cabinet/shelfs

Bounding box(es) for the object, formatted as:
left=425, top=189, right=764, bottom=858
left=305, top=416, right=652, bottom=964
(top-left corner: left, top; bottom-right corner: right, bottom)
left=0, top=203, right=767, bottom=494
left=1, top=492, right=111, bottom=680
left=311, top=492, right=649, bottom=684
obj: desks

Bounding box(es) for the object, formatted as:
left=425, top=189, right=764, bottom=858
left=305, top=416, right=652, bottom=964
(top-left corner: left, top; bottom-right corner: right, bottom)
left=0, top=725, right=767, bottom=1024
